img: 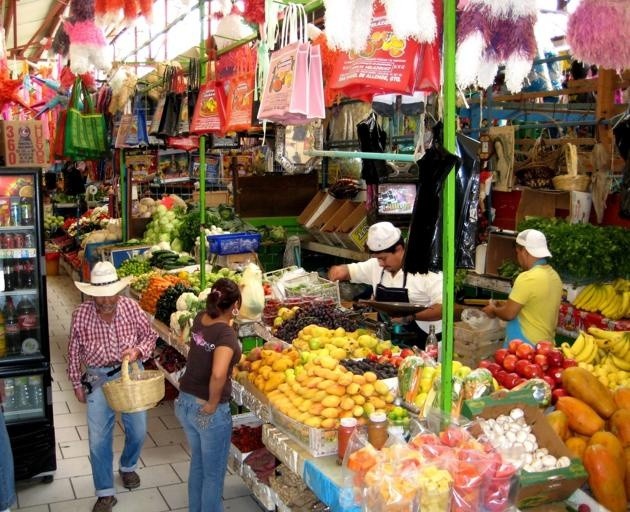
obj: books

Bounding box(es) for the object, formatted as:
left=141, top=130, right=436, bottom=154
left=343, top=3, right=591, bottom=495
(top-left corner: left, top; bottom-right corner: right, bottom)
left=0, top=121, right=48, bottom=167
left=513, top=184, right=592, bottom=232
left=296, top=188, right=373, bottom=253
left=485, top=231, right=522, bottom=280
left=459, top=390, right=590, bottom=509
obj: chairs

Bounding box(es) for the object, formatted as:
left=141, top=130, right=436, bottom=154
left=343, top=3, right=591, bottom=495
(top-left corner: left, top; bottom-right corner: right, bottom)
left=93, top=495, right=117, bottom=512
left=120, top=468, right=141, bottom=489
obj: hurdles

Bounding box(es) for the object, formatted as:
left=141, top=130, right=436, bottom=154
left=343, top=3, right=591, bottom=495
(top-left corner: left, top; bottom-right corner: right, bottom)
left=248, top=349, right=300, bottom=392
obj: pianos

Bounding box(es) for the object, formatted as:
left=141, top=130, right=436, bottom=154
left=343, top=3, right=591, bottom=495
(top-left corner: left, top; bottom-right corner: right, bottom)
left=140, top=275, right=190, bottom=314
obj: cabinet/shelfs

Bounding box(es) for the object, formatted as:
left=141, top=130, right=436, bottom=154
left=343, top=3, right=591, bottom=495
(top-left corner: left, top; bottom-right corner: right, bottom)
left=0, top=294, right=38, bottom=357
left=1, top=374, right=42, bottom=411
left=425, top=325, right=439, bottom=361
left=367, top=411, right=389, bottom=451
left=337, top=417, right=357, bottom=459
left=4, top=256, right=35, bottom=290
left=412, top=345, right=434, bottom=365
left=11, top=195, right=32, bottom=225
left=375, top=322, right=387, bottom=344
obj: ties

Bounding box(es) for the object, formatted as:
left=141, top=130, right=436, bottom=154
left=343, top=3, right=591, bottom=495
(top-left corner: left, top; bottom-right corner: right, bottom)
left=376, top=182, right=418, bottom=226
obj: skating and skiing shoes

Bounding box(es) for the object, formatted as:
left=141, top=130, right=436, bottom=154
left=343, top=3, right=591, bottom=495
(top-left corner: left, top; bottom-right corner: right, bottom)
left=274, top=304, right=360, bottom=343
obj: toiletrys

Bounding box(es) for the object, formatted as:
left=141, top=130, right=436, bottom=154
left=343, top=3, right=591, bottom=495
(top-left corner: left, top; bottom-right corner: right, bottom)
left=116, top=258, right=151, bottom=276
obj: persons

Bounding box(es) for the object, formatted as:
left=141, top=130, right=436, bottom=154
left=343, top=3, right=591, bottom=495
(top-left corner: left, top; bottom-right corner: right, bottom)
left=482, top=229, right=562, bottom=352
left=0, top=378, right=17, bottom=512
left=66, top=261, right=157, bottom=512
left=175, top=279, right=242, bottom=512
left=328, top=221, right=444, bottom=360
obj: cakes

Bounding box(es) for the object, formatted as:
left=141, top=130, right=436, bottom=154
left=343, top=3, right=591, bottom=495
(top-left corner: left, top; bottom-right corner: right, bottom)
left=0, top=232, right=32, bottom=248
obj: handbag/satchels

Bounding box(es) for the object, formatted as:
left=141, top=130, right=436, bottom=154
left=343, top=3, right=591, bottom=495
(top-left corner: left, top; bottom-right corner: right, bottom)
left=413, top=313, right=417, bottom=320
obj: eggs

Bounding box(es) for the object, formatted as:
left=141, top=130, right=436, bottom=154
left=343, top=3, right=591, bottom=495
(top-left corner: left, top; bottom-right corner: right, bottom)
left=127, top=204, right=182, bottom=251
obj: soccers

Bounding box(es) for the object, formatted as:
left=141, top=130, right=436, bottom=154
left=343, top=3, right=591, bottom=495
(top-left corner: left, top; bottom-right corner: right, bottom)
left=366, top=349, right=415, bottom=369
left=477, top=340, right=578, bottom=404
left=286, top=324, right=393, bottom=381
left=415, top=362, right=471, bottom=409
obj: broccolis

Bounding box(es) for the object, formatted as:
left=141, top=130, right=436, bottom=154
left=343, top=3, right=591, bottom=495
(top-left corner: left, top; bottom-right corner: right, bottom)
left=0, top=167, right=56, bottom=489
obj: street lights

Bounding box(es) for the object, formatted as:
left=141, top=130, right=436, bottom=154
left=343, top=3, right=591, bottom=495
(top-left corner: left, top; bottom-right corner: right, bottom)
left=328, top=1, right=445, bottom=99
left=255, top=42, right=327, bottom=124
left=54, top=109, right=110, bottom=163
left=114, top=70, right=256, bottom=154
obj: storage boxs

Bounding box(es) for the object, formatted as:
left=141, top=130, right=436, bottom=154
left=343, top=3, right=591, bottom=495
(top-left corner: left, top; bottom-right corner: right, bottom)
left=252, top=473, right=275, bottom=510
left=230, top=379, right=244, bottom=405
left=161, top=335, right=190, bottom=358
left=227, top=411, right=261, bottom=477
left=296, top=188, right=373, bottom=253
left=155, top=360, right=186, bottom=389
left=0, top=121, right=48, bottom=167
left=513, top=184, right=592, bottom=232
left=242, top=389, right=270, bottom=423
left=459, top=390, right=590, bottom=509
left=243, top=458, right=263, bottom=488
left=485, top=231, right=522, bottom=280
left=262, top=409, right=362, bottom=510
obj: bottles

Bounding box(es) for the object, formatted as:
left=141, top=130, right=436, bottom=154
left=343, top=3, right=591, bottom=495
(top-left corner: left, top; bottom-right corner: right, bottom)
left=75, top=261, right=133, bottom=297
left=516, top=229, right=553, bottom=258
left=366, top=221, right=402, bottom=252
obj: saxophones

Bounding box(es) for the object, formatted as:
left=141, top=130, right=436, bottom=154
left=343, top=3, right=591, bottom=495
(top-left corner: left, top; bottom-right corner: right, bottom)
left=479, top=408, right=571, bottom=473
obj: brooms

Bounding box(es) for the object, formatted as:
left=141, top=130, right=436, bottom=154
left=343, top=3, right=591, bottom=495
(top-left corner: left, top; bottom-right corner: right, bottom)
left=543, top=366, right=630, bottom=512
left=267, top=348, right=394, bottom=439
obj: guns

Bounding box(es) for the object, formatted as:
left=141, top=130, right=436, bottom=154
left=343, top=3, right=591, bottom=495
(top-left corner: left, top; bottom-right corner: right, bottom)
left=561, top=328, right=630, bottom=373
left=572, top=277, right=630, bottom=320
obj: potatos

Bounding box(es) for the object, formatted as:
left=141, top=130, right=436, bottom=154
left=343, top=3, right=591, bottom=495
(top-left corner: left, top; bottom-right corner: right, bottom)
left=149, top=250, right=195, bottom=269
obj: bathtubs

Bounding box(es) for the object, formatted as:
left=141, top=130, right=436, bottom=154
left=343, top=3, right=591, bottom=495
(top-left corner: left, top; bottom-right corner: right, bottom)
left=170, top=288, right=211, bottom=336
